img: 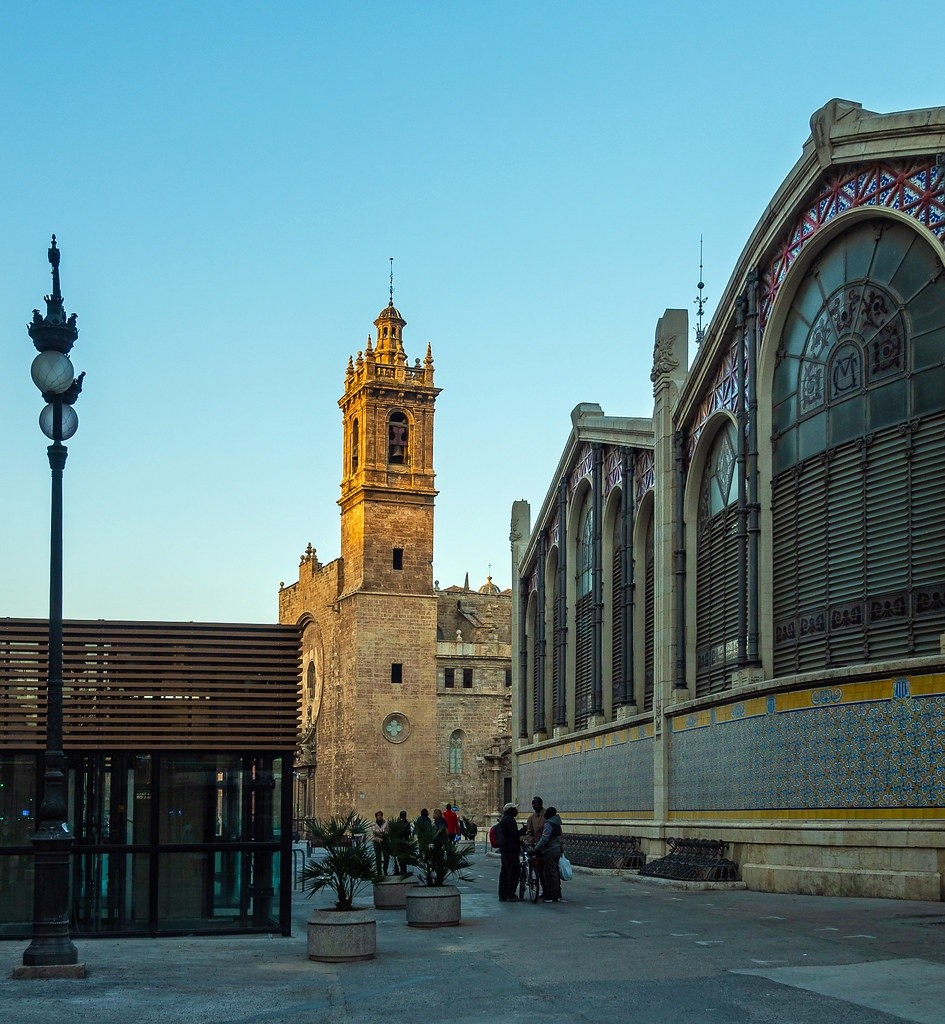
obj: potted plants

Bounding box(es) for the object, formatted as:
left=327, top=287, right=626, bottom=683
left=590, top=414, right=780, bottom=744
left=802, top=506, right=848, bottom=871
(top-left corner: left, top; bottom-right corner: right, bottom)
left=372, top=815, right=419, bottom=910
left=296, top=809, right=382, bottom=962
left=406, top=817, right=479, bottom=927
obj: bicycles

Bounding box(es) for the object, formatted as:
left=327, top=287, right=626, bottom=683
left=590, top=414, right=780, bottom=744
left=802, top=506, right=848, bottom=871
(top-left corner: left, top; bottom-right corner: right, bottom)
left=517, top=839, right=541, bottom=904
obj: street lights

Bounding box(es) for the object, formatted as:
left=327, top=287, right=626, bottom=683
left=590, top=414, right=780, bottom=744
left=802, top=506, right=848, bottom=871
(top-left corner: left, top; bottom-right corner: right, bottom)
left=23, top=234, right=80, bottom=964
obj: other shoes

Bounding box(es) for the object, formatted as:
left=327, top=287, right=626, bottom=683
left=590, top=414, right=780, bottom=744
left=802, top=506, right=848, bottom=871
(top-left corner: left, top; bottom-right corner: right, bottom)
left=498, top=894, right=518, bottom=902
left=384, top=867, right=387, bottom=875
left=543, top=896, right=561, bottom=902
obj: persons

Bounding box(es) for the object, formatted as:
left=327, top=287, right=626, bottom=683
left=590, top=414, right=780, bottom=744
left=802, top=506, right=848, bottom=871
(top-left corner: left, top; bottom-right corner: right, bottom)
left=372, top=804, right=477, bottom=878
left=496, top=796, right=563, bottom=903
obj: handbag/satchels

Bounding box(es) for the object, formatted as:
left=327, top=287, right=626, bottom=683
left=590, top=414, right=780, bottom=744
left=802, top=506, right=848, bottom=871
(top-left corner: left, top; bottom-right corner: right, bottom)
left=380, top=834, right=388, bottom=847
left=454, top=835, right=461, bottom=841
left=558, top=854, right=572, bottom=881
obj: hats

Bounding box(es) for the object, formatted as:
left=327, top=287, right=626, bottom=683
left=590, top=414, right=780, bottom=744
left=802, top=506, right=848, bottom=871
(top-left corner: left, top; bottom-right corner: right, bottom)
left=502, top=802, right=520, bottom=812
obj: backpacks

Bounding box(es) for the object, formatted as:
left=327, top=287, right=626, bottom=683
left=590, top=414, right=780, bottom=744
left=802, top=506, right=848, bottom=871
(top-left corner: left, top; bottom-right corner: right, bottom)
left=489, top=817, right=510, bottom=848
